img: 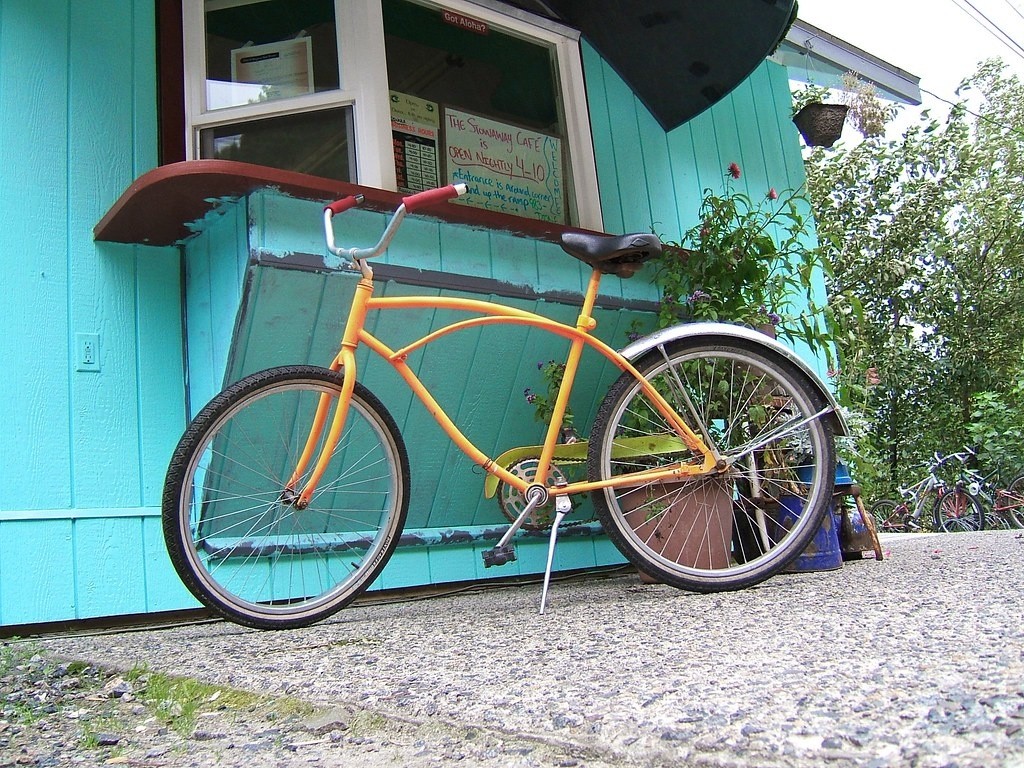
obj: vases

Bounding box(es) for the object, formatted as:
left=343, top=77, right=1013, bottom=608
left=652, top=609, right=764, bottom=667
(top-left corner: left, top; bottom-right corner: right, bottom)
left=622, top=468, right=736, bottom=582
left=791, top=102, right=850, bottom=146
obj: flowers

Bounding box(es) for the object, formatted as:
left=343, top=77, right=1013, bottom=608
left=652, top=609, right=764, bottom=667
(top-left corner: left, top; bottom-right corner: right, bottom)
left=788, top=68, right=886, bottom=137
left=521, top=161, right=880, bottom=533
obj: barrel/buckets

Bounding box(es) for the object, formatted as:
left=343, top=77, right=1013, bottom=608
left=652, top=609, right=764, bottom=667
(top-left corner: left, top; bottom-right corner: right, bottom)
left=774, top=493, right=843, bottom=571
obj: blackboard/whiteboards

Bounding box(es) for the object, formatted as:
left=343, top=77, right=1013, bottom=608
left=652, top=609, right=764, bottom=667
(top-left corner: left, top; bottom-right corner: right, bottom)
left=439, top=102, right=571, bottom=224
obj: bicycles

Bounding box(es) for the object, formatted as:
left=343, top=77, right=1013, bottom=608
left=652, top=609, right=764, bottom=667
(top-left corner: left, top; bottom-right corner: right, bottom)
left=871, top=443, right=1024, bottom=532
left=162, top=181, right=852, bottom=629
